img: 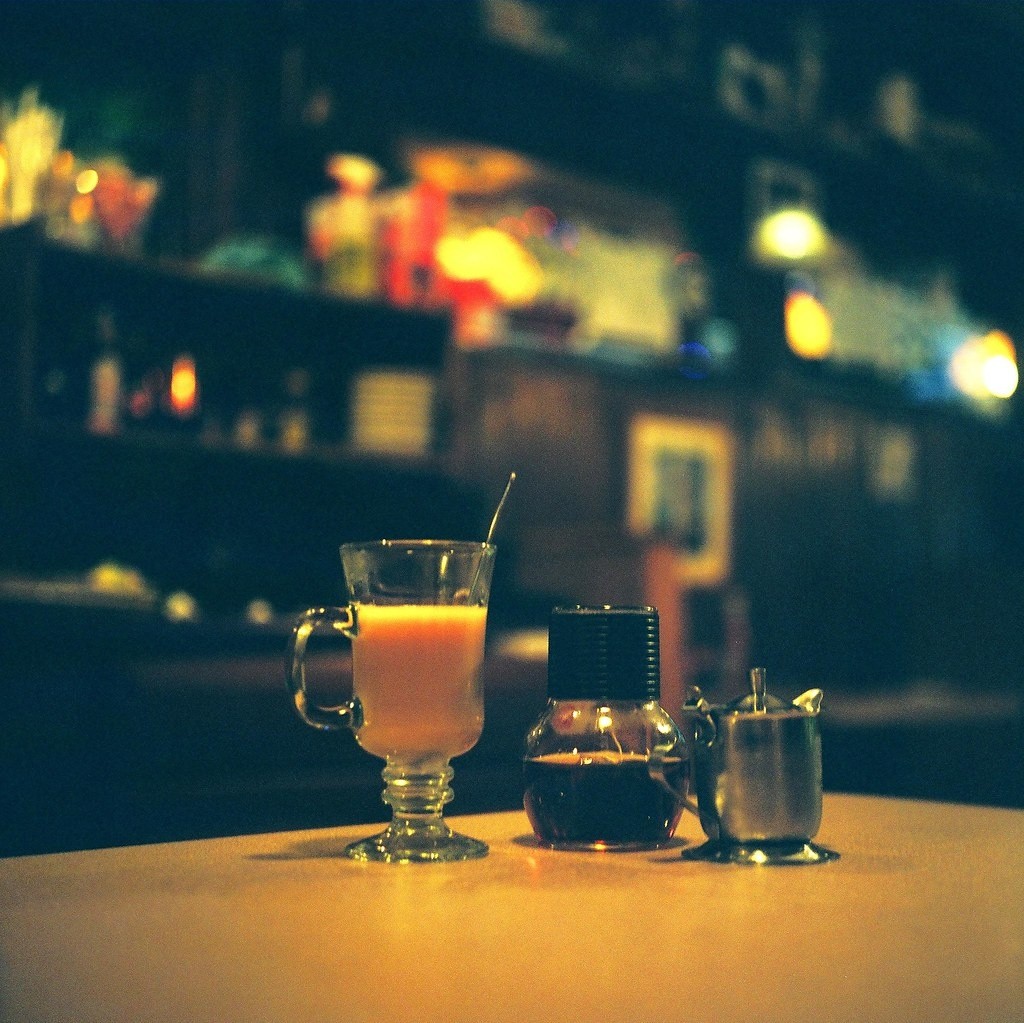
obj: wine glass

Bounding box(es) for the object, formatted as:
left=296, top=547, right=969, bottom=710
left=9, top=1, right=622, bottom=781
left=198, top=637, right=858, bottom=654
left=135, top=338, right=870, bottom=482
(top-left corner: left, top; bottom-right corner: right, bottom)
left=287, top=540, right=497, bottom=864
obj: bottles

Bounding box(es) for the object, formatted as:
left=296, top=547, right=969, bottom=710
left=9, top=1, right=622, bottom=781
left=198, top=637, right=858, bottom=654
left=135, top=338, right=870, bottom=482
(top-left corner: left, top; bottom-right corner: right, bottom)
left=520, top=603, right=688, bottom=854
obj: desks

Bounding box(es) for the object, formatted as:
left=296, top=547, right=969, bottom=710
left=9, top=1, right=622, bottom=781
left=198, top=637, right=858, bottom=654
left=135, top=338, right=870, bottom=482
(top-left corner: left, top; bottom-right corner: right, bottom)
left=0, top=793, right=1024, bottom=1023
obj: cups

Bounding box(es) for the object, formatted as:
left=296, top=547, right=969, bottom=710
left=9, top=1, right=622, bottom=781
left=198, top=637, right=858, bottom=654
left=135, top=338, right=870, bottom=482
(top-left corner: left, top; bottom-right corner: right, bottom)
left=647, top=667, right=840, bottom=866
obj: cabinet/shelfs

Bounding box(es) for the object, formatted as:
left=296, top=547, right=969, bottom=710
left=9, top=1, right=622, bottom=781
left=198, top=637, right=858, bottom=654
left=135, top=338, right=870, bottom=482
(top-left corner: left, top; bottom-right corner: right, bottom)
left=0, top=215, right=480, bottom=857
